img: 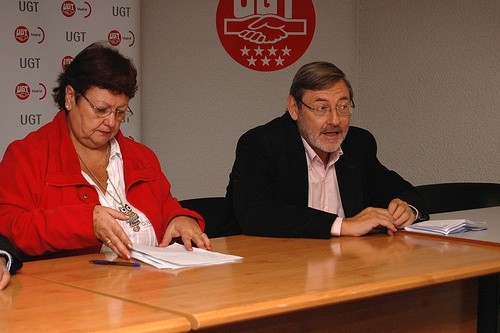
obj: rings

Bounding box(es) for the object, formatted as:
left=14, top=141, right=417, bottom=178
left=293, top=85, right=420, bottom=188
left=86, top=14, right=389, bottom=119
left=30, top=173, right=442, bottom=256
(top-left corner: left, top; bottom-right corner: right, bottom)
left=199, top=232, right=206, bottom=237
left=105, top=239, right=111, bottom=244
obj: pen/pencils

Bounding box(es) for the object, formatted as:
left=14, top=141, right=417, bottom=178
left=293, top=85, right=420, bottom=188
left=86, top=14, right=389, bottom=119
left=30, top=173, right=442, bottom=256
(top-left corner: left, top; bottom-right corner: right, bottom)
left=88, top=260, right=141, bottom=267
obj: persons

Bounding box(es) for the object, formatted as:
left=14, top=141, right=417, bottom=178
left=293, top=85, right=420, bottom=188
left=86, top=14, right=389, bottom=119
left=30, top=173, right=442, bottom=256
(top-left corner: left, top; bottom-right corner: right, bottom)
left=211, top=61, right=439, bottom=240
left=0, top=233, right=24, bottom=290
left=0, top=43, right=212, bottom=260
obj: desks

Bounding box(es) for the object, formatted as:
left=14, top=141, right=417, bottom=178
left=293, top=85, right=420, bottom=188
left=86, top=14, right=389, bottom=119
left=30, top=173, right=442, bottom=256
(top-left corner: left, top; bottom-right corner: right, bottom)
left=0, top=271, right=192, bottom=333
left=15, top=228, right=500, bottom=333
left=402, top=205, right=500, bottom=244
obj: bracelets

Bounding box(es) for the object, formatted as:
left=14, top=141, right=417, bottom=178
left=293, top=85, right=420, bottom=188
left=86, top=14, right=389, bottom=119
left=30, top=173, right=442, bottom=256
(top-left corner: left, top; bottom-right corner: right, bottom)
left=409, top=206, right=418, bottom=216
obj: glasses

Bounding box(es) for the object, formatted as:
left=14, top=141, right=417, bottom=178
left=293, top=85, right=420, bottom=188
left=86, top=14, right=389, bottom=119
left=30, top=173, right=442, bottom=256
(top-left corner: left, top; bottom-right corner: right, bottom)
left=295, top=97, right=355, bottom=117
left=80, top=91, right=134, bottom=123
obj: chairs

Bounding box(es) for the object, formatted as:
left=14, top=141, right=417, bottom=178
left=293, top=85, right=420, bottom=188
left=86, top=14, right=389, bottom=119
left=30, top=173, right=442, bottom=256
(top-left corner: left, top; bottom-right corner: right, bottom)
left=413, top=182, right=500, bottom=216
left=178, top=196, right=228, bottom=247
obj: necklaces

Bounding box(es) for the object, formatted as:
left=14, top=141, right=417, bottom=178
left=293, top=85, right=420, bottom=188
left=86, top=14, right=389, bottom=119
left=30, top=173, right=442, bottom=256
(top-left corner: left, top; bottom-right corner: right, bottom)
left=76, top=140, right=140, bottom=232
